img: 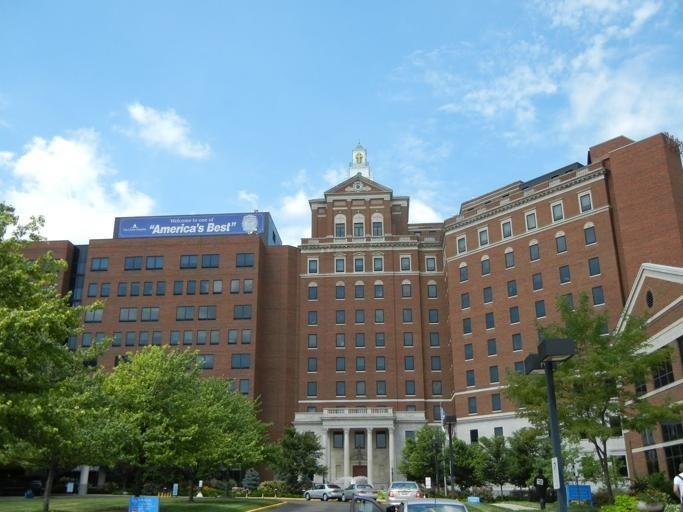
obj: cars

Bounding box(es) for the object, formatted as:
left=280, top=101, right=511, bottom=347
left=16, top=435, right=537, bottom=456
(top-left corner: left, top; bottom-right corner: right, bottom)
left=302, top=480, right=468, bottom=511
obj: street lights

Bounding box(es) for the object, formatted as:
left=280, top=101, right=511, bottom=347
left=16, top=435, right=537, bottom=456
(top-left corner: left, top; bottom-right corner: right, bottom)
left=441, top=414, right=459, bottom=499
left=523, top=338, right=580, bottom=510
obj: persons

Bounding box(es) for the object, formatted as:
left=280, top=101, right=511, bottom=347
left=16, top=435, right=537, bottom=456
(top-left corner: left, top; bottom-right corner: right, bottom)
left=394, top=505, right=400, bottom=512
left=534, top=468, right=548, bottom=509
left=673, top=462, right=683, bottom=512
